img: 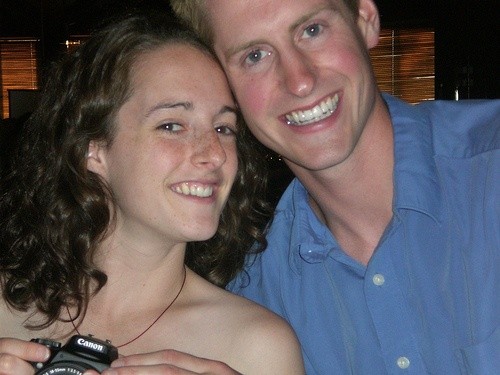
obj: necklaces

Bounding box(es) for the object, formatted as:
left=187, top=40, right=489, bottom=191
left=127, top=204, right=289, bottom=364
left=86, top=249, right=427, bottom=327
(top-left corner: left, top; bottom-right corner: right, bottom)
left=62, top=261, right=187, bottom=348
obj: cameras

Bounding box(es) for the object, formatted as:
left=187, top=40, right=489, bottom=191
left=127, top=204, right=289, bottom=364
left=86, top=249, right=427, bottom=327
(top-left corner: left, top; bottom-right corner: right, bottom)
left=24, top=335, right=119, bottom=375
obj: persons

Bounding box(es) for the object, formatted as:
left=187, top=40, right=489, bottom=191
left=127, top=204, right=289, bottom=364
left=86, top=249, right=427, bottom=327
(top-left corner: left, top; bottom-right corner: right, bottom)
left=166, top=0, right=499, bottom=375
left=0, top=18, right=307, bottom=373
